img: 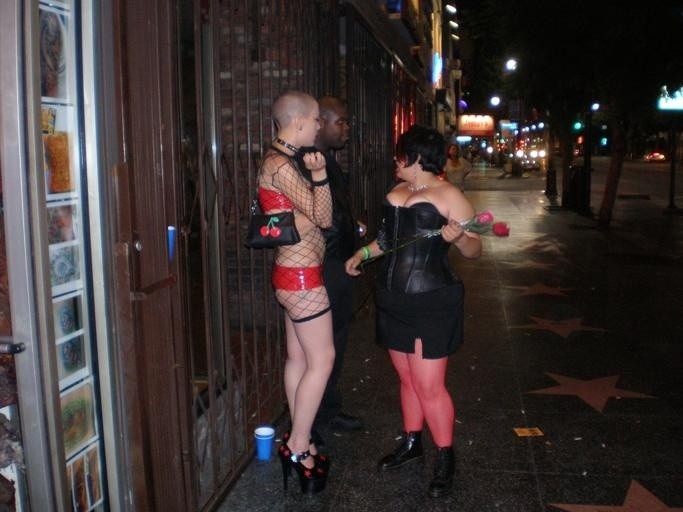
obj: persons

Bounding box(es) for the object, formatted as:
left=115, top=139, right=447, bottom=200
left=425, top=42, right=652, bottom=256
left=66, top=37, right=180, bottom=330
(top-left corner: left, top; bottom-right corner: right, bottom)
left=256, top=89, right=338, bottom=495
left=306, top=96, right=367, bottom=447
left=343, top=124, right=481, bottom=497
left=443, top=144, right=472, bottom=193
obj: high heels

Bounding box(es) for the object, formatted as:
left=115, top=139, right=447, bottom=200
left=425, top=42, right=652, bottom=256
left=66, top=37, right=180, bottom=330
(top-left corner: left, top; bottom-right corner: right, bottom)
left=276, top=430, right=330, bottom=495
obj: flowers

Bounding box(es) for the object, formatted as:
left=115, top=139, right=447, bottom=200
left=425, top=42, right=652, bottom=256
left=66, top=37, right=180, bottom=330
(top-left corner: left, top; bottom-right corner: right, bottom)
left=362, top=211, right=509, bottom=266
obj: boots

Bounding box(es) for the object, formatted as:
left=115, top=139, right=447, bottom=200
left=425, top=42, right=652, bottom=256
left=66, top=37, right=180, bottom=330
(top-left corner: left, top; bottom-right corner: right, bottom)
left=428, top=446, right=460, bottom=500
left=378, top=430, right=426, bottom=470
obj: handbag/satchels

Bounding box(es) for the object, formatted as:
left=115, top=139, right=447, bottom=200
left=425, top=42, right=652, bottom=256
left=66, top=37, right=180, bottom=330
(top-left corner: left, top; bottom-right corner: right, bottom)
left=244, top=211, right=301, bottom=250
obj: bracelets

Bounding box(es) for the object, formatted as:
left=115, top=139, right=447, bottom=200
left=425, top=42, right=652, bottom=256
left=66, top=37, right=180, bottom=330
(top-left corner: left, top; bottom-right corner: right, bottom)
left=312, top=177, right=329, bottom=186
left=361, top=246, right=370, bottom=260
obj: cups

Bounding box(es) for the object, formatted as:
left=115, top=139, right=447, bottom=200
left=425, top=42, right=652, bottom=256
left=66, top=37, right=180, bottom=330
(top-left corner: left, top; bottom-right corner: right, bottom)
left=168, top=226, right=176, bottom=264
left=254, top=427, right=275, bottom=462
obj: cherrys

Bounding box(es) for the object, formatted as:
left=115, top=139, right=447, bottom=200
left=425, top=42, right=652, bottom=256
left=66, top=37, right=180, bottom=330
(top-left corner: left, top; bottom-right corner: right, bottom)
left=260, top=217, right=282, bottom=238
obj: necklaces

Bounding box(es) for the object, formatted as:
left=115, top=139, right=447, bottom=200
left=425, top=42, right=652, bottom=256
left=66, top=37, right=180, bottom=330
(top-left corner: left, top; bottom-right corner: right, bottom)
left=408, top=183, right=429, bottom=192
left=274, top=137, right=300, bottom=153
